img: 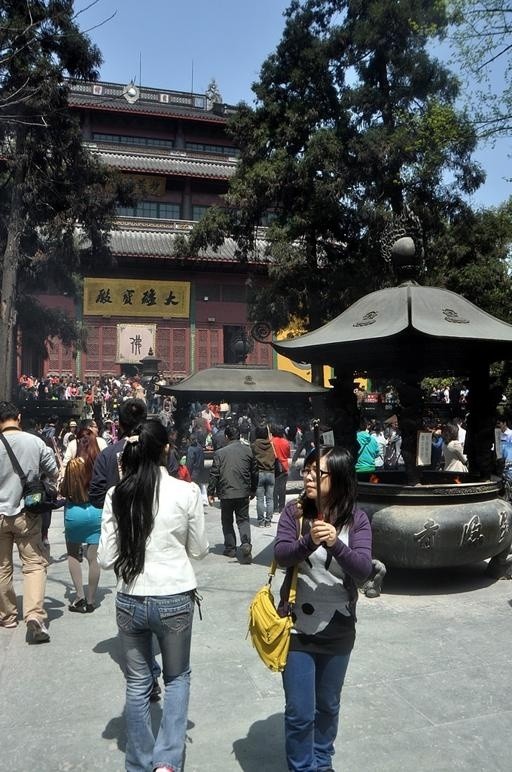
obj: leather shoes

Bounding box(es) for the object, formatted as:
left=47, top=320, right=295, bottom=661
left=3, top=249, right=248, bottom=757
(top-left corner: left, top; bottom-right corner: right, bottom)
left=69, top=598, right=94, bottom=612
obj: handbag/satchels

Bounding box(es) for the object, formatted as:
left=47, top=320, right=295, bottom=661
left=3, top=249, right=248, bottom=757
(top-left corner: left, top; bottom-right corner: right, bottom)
left=249, top=584, right=293, bottom=672
left=275, top=458, right=285, bottom=478
left=23, top=480, right=58, bottom=513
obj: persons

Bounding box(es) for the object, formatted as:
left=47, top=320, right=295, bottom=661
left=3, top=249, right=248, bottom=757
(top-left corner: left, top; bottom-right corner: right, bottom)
left=16, top=372, right=186, bottom=561
left=356, top=418, right=404, bottom=472
left=184, top=403, right=314, bottom=565
left=89, top=397, right=180, bottom=700
left=269, top=444, right=372, bottom=771
left=496, top=415, right=512, bottom=504
left=431, top=385, right=469, bottom=403
left=56, top=428, right=105, bottom=615
left=356, top=385, right=398, bottom=403
left=0, top=401, right=61, bottom=645
left=424, top=414, right=470, bottom=472
left=95, top=420, right=210, bottom=772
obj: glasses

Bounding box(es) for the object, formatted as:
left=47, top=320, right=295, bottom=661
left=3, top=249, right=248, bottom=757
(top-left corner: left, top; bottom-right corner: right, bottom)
left=300, top=469, right=329, bottom=478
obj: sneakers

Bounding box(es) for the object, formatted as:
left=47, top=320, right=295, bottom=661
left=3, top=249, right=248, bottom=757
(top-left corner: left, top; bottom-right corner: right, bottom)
left=26, top=618, right=50, bottom=643
